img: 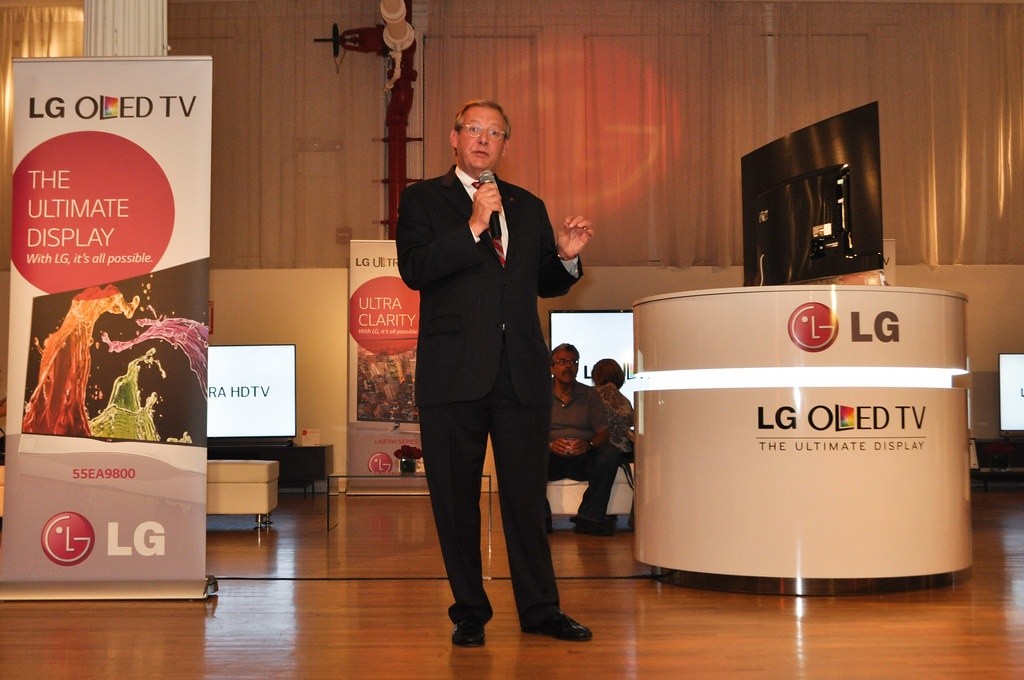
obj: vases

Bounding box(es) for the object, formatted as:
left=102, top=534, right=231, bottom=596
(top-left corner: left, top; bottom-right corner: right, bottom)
left=399, top=459, right=416, bottom=472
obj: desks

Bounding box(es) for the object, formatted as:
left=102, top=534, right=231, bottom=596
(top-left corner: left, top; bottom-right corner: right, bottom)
left=208, top=445, right=335, bottom=498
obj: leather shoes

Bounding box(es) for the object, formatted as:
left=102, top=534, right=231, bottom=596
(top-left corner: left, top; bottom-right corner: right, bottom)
left=452, top=617, right=486, bottom=646
left=521, top=610, right=592, bottom=642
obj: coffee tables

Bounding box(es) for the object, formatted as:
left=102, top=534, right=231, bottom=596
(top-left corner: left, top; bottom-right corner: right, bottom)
left=326, top=472, right=492, bottom=555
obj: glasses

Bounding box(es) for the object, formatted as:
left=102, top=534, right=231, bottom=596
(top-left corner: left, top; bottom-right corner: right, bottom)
left=458, top=123, right=506, bottom=142
left=553, top=358, right=579, bottom=367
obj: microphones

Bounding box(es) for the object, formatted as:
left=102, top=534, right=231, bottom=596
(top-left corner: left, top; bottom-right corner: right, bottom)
left=479, top=170, right=501, bottom=238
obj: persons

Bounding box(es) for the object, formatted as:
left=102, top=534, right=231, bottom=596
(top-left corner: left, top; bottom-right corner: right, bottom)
left=396, top=100, right=594, bottom=649
left=591, top=359, right=635, bottom=463
left=543, top=344, right=620, bottom=536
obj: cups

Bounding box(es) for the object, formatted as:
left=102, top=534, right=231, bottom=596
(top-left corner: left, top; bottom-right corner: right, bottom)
left=563, top=438, right=576, bottom=455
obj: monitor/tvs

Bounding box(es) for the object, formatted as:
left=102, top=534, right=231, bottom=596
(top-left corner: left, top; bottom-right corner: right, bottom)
left=739, top=100, right=883, bottom=288
left=356, top=338, right=421, bottom=435
left=998, top=352, right=1024, bottom=437
left=548, top=309, right=635, bottom=433
left=18, top=257, right=209, bottom=474
left=208, top=344, right=297, bottom=446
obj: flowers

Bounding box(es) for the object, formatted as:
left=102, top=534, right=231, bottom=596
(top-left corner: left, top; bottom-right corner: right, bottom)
left=393, top=445, right=422, bottom=460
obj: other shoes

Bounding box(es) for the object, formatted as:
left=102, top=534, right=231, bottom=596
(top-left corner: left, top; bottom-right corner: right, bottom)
left=575, top=517, right=616, bottom=536
left=545, top=519, right=554, bottom=536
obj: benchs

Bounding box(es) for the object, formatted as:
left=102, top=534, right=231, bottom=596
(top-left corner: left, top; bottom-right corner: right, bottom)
left=207, top=460, right=280, bottom=532
left=969, top=439, right=1023, bottom=492
left=544, top=464, right=638, bottom=515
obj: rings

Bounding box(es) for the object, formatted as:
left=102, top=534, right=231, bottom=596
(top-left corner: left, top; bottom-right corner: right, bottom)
left=583, top=227, right=587, bottom=230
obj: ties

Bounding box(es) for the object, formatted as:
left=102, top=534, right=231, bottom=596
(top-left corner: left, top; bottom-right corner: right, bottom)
left=472, top=181, right=505, bottom=268
